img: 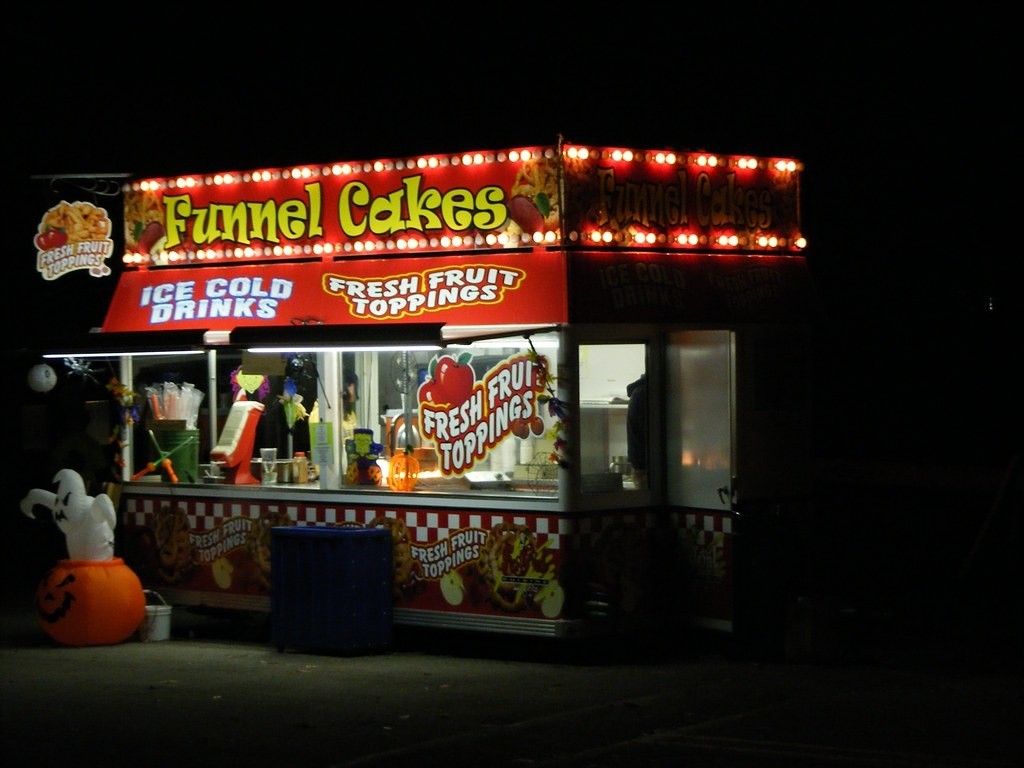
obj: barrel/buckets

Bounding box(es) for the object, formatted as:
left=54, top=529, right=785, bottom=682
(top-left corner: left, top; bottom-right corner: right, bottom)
left=156, top=429, right=201, bottom=485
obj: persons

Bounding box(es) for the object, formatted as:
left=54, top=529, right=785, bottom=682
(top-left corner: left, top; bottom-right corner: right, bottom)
left=308, top=370, right=361, bottom=446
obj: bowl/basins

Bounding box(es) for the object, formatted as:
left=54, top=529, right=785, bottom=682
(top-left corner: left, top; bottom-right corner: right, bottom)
left=202, top=476, right=225, bottom=485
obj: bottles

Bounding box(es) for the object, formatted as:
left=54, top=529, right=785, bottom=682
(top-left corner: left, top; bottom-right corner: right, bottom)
left=291, top=452, right=309, bottom=483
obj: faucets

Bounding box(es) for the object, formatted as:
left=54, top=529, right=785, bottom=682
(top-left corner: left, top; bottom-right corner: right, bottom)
left=609, top=461, right=635, bottom=482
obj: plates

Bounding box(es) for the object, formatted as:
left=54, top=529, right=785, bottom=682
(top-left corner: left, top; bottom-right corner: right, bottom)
left=463, top=472, right=512, bottom=489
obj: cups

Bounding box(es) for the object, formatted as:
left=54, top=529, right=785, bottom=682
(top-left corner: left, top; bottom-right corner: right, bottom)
left=503, top=436, right=536, bottom=472
left=259, top=448, right=280, bottom=485
